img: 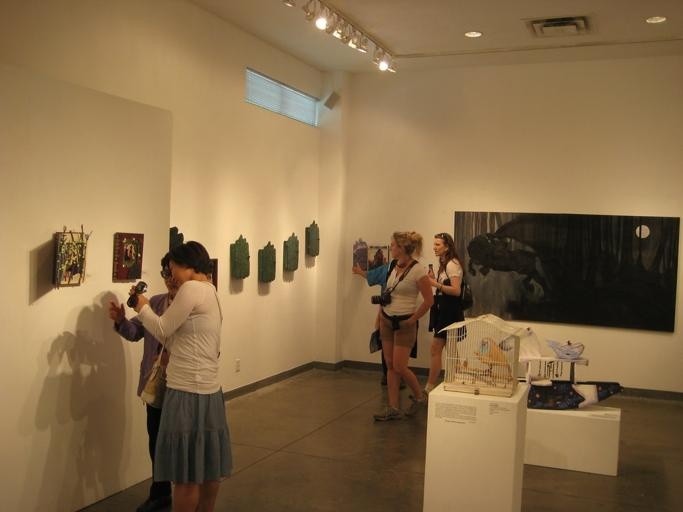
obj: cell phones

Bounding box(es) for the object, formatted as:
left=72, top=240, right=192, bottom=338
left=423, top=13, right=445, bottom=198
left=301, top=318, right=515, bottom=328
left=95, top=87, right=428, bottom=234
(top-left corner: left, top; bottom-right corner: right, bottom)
left=429, top=265, right=433, bottom=274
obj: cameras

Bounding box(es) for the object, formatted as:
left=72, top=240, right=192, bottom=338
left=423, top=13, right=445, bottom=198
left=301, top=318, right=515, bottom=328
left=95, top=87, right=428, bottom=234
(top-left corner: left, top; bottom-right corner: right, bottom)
left=135, top=281, right=147, bottom=294
left=372, top=293, right=391, bottom=304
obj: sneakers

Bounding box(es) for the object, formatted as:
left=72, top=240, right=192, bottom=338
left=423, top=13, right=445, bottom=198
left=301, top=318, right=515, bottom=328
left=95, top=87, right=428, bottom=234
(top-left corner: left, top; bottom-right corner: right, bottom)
left=404, top=390, right=428, bottom=416
left=136, top=496, right=172, bottom=512
left=381, top=375, right=406, bottom=390
left=373, top=405, right=403, bottom=421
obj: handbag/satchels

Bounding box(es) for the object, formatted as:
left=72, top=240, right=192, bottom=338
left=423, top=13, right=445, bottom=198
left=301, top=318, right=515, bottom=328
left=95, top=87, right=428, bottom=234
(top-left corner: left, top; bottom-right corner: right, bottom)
left=140, top=361, right=167, bottom=408
left=460, top=279, right=473, bottom=310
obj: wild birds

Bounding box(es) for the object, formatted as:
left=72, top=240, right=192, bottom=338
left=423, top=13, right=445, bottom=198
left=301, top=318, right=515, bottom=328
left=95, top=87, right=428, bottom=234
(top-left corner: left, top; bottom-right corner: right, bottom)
left=480, top=338, right=513, bottom=374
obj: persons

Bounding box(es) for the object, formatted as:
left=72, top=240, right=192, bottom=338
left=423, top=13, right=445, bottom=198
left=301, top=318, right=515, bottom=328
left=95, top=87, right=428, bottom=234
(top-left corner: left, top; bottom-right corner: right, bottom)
left=123, top=239, right=229, bottom=510
left=352, top=262, right=419, bottom=390
left=107, top=251, right=180, bottom=511
left=407, top=232, right=474, bottom=401
left=372, top=230, right=426, bottom=424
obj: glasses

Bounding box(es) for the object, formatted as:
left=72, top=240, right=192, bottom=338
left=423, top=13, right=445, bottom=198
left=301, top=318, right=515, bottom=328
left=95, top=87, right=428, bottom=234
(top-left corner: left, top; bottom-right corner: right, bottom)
left=160, top=270, right=172, bottom=279
left=127, top=281, right=147, bottom=308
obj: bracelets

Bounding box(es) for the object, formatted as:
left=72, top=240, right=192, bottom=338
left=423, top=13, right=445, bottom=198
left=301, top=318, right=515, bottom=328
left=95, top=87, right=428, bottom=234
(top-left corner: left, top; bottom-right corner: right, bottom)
left=439, top=285, right=443, bottom=292
